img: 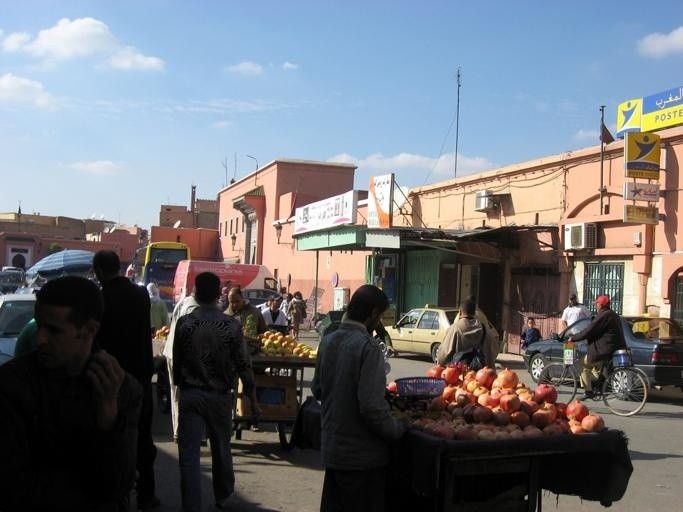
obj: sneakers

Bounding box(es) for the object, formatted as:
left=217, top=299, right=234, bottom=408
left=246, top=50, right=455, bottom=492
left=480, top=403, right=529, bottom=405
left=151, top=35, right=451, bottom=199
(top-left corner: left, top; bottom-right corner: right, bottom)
left=578, top=392, right=593, bottom=400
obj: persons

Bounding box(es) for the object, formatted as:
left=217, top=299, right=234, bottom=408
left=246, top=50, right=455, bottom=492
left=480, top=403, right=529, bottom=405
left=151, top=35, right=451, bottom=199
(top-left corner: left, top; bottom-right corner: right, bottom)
left=84, top=249, right=162, bottom=512
left=568, top=295, right=628, bottom=401
left=435, top=294, right=488, bottom=369
left=561, top=293, right=591, bottom=332
left=519, top=316, right=543, bottom=353
left=1, top=276, right=145, bottom=512
left=172, top=270, right=255, bottom=512
left=310, top=284, right=406, bottom=512
left=147, top=281, right=170, bottom=338
left=162, top=286, right=200, bottom=444
left=216, top=283, right=307, bottom=339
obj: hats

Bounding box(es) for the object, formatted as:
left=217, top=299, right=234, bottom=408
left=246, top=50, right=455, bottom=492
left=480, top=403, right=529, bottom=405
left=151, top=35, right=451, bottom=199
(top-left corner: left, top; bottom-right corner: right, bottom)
left=592, top=295, right=610, bottom=307
left=569, top=294, right=576, bottom=299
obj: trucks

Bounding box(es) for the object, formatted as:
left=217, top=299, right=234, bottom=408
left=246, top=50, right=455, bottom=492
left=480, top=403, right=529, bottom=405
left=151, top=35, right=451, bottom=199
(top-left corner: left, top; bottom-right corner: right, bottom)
left=171, top=258, right=284, bottom=323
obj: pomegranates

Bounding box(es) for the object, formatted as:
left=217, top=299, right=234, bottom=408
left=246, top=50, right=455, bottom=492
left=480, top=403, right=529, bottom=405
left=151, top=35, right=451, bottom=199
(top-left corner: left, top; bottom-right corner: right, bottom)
left=388, top=362, right=605, bottom=442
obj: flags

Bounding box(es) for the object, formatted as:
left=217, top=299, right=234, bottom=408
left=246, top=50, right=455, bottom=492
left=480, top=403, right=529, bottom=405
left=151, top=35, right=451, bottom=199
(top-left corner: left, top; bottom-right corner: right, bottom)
left=599, top=118, right=615, bottom=145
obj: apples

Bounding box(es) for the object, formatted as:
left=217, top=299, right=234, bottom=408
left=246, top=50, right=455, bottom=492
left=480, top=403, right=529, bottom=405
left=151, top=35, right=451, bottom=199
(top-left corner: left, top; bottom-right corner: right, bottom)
left=257, top=330, right=317, bottom=359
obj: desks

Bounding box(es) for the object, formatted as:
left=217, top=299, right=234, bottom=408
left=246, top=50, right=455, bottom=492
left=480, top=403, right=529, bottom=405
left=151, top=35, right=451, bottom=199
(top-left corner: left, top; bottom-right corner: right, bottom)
left=230, top=352, right=321, bottom=451
left=298, top=383, right=631, bottom=512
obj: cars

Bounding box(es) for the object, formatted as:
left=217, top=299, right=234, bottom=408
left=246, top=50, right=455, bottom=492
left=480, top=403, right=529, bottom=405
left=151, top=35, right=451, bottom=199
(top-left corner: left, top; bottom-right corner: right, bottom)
left=218, top=288, right=285, bottom=315
left=522, top=316, right=683, bottom=401
left=373, top=305, right=499, bottom=367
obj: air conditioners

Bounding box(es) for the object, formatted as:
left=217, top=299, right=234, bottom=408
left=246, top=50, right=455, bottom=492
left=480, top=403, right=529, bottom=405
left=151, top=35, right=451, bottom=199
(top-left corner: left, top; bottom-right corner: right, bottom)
left=560, top=219, right=599, bottom=253
left=472, top=189, right=494, bottom=212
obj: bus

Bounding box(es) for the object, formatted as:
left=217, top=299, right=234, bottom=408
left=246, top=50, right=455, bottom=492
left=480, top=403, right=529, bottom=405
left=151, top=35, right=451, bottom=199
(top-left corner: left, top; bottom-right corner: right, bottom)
left=132, top=238, right=191, bottom=305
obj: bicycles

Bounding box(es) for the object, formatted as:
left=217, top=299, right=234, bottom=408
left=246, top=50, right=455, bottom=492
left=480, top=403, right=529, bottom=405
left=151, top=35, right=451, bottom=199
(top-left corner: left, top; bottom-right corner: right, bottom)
left=537, top=332, right=648, bottom=417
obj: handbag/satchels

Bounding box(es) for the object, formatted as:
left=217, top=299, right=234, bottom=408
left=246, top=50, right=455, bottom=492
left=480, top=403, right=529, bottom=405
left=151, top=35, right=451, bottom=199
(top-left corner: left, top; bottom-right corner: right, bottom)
left=450, top=347, right=486, bottom=371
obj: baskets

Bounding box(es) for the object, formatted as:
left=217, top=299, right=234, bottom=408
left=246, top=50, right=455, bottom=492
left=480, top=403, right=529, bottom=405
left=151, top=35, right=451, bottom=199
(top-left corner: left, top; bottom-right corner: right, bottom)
left=396, top=377, right=446, bottom=398
